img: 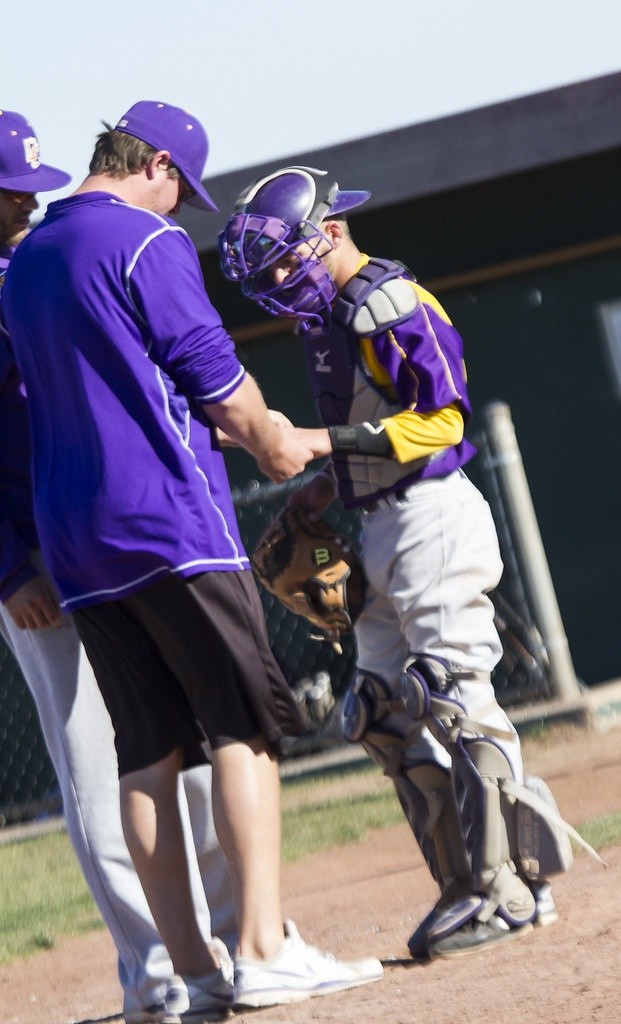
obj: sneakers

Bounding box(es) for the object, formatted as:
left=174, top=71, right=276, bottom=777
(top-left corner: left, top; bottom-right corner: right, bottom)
left=161, top=937, right=235, bottom=1024
left=231, top=918, right=384, bottom=1007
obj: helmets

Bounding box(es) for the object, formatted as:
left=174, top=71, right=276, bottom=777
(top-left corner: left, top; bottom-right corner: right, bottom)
left=245, top=172, right=373, bottom=239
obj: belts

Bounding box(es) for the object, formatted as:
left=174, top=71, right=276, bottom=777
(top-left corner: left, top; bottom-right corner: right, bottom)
left=364, top=490, right=405, bottom=514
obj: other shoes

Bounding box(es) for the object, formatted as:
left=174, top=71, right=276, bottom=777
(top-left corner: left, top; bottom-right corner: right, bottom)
left=124, top=1001, right=179, bottom=1024
left=428, top=910, right=533, bottom=963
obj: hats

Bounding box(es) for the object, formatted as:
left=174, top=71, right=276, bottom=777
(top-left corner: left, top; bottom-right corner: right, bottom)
left=116, top=100, right=221, bottom=213
left=0, top=110, right=71, bottom=193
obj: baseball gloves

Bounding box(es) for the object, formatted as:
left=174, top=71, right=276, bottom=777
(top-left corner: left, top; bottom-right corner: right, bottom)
left=248, top=501, right=357, bottom=630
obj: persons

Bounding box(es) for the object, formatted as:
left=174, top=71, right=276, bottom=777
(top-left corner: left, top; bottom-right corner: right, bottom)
left=215, top=164, right=560, bottom=963
left=0, top=99, right=384, bottom=1010
left=0, top=108, right=243, bottom=1024
